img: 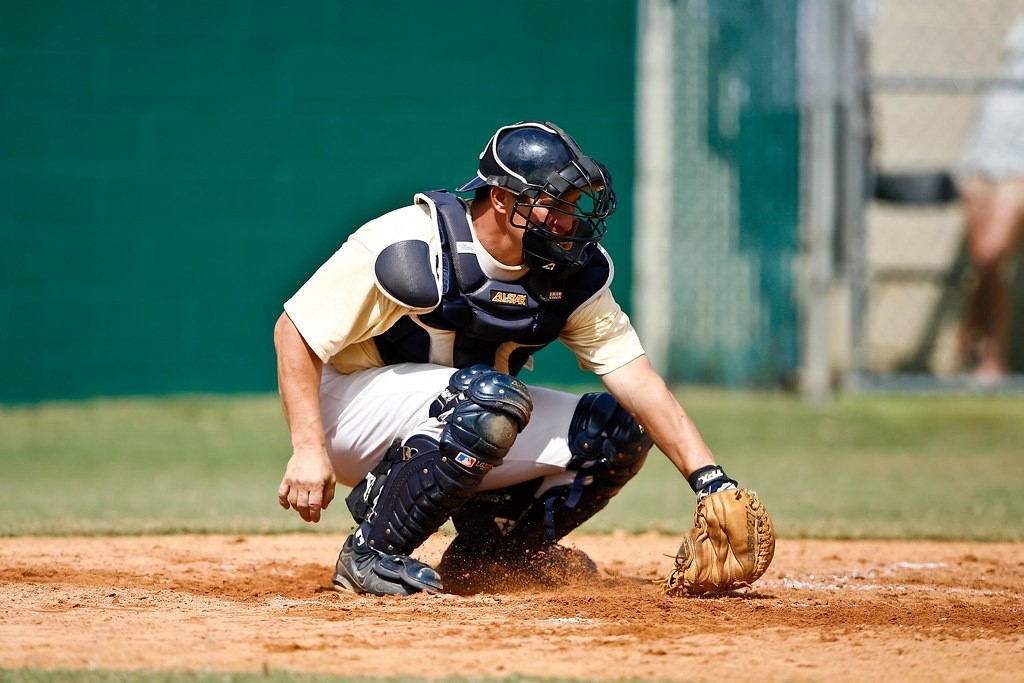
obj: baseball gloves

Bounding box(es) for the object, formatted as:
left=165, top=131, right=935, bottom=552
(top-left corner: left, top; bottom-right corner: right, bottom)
left=663, top=487, right=775, bottom=598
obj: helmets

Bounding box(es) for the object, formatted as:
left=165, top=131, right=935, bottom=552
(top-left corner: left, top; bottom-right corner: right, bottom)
left=454, top=120, right=604, bottom=199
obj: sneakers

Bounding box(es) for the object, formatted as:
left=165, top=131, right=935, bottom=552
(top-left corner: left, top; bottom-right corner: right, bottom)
left=330, top=535, right=444, bottom=597
left=436, top=536, right=597, bottom=587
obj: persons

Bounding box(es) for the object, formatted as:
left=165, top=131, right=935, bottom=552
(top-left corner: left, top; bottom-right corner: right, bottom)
left=949, top=15, right=1024, bottom=378
left=274, top=120, right=763, bottom=597
